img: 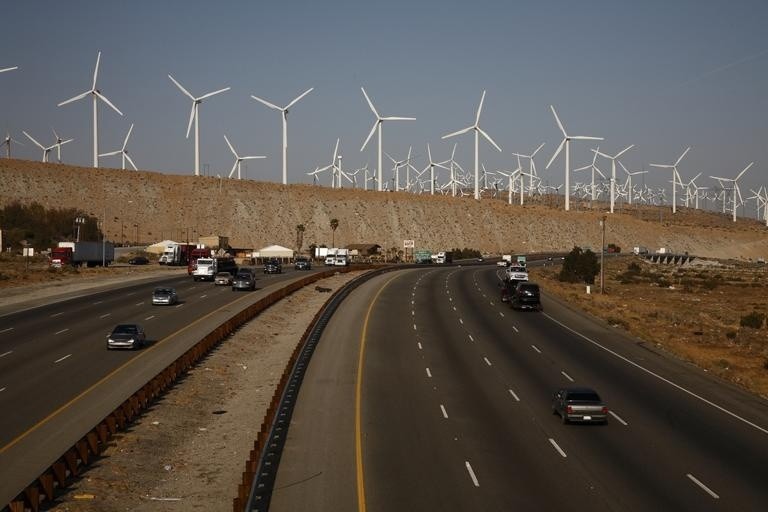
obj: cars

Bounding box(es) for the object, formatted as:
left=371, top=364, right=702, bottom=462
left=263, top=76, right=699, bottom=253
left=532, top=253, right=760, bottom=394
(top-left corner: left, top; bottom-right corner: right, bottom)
left=263, top=261, right=281, bottom=274
left=104, top=323, right=145, bottom=351
left=496, top=254, right=543, bottom=313
left=128, top=256, right=150, bottom=265
left=214, top=271, right=234, bottom=286
left=232, top=273, right=256, bottom=292
left=294, top=258, right=311, bottom=271
left=152, top=287, right=178, bottom=306
left=551, top=386, right=609, bottom=425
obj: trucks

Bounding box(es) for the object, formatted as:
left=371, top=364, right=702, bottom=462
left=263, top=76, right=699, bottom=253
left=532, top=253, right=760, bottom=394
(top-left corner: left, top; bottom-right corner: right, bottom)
left=437, top=252, right=453, bottom=264
left=48, top=240, right=114, bottom=268
left=634, top=246, right=648, bottom=256
left=188, top=248, right=239, bottom=282
left=324, top=248, right=349, bottom=267
left=158, top=244, right=197, bottom=266
